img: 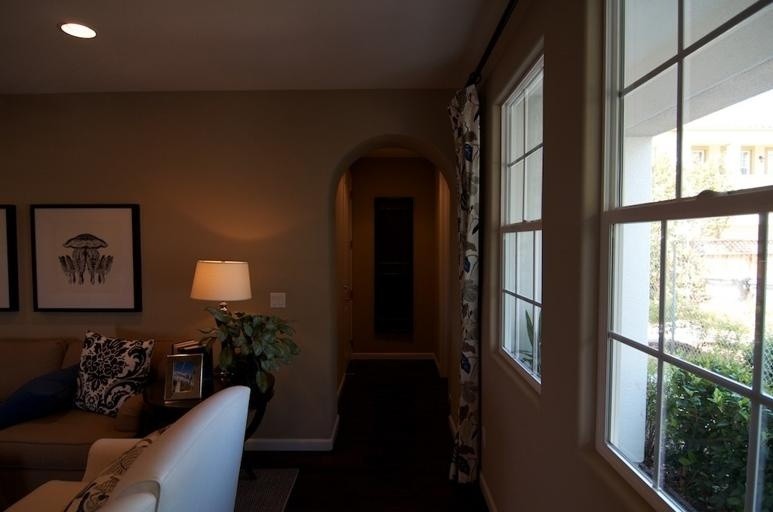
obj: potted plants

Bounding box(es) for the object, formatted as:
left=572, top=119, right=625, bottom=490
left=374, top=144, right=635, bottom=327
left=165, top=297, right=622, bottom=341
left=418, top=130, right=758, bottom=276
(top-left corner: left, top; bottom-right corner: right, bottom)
left=202, top=307, right=302, bottom=389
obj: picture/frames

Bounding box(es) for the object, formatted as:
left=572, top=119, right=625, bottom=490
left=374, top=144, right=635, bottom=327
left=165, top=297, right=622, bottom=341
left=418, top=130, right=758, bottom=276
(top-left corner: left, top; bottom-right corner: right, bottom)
left=0, top=204, right=143, bottom=314
left=162, top=340, right=213, bottom=404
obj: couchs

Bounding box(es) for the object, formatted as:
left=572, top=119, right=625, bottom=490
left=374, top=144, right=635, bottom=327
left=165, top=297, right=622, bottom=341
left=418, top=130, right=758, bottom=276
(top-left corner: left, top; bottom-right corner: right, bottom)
left=0, top=337, right=147, bottom=510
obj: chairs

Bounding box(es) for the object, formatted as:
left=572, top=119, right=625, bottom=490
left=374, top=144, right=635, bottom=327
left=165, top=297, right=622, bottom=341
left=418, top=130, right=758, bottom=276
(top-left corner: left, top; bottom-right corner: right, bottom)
left=1, top=384, right=253, bottom=511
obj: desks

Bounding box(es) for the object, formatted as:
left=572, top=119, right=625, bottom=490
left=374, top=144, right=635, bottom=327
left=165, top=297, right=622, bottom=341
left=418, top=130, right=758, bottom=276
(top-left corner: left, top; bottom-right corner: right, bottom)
left=144, top=369, right=276, bottom=482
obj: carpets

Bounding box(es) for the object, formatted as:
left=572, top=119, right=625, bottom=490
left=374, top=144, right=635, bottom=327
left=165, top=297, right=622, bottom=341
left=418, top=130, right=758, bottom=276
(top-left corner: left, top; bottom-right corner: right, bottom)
left=233, top=468, right=301, bottom=512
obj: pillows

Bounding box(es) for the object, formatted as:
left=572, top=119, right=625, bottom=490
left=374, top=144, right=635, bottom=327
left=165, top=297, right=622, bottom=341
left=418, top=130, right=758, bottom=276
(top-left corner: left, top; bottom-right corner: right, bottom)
left=64, top=418, right=173, bottom=512
left=0, top=330, right=156, bottom=431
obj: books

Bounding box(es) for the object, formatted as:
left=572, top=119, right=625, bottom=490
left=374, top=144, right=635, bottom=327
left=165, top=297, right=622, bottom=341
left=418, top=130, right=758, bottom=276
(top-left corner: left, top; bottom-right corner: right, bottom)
left=171, top=338, right=215, bottom=398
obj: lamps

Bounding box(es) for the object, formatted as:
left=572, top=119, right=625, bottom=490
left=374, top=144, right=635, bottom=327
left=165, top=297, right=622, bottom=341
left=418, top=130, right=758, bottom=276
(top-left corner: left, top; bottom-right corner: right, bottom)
left=189, top=259, right=252, bottom=385
